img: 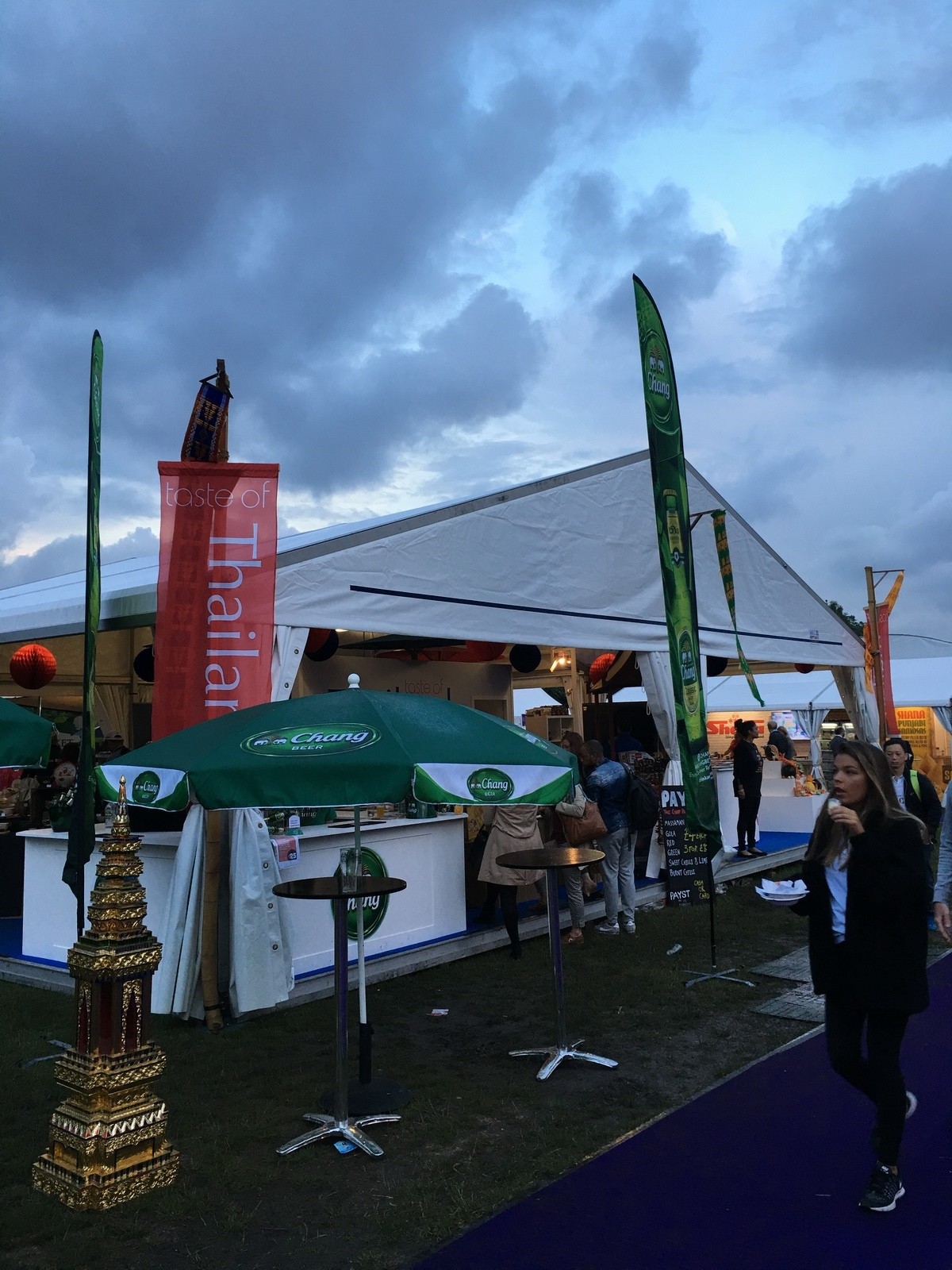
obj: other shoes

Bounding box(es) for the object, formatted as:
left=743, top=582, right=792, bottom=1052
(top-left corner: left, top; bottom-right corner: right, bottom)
left=737, top=851, right=756, bottom=858
left=749, top=850, right=767, bottom=856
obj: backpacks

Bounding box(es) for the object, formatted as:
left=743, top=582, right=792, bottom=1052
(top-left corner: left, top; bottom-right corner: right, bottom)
left=620, top=763, right=660, bottom=830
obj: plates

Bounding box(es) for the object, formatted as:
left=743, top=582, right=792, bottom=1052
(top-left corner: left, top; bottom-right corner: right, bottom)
left=760, top=889, right=809, bottom=906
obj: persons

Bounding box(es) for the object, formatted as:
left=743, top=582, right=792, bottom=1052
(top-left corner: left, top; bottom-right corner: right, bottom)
left=476, top=718, right=858, bottom=961
left=0, top=769, right=22, bottom=791
left=105, top=730, right=131, bottom=761
left=802, top=741, right=933, bottom=1212
left=933, top=779, right=952, bottom=943
left=881, top=737, right=941, bottom=841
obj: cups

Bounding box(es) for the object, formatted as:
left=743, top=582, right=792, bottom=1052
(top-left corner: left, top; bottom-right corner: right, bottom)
left=377, top=806, right=384, bottom=817
left=454, top=805, right=463, bottom=815
left=340, top=847, right=359, bottom=892
left=18, top=801, right=28, bottom=815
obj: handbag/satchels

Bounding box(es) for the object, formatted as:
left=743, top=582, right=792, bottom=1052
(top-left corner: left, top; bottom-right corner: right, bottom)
left=558, top=787, right=607, bottom=845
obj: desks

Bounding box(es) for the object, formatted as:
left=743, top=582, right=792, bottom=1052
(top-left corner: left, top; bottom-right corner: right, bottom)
left=273, top=877, right=407, bottom=1158
left=495, top=847, right=618, bottom=1082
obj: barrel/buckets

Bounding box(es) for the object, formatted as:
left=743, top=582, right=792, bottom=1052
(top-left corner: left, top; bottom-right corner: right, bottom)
left=405, top=792, right=437, bottom=819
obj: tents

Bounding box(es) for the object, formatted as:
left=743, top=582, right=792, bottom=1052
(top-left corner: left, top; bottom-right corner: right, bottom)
left=0, top=448, right=952, bottom=987
left=506, top=654, right=952, bottom=787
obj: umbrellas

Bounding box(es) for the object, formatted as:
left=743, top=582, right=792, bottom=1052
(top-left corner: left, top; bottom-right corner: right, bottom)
left=94, top=687, right=580, bottom=1122
left=0, top=697, right=51, bottom=768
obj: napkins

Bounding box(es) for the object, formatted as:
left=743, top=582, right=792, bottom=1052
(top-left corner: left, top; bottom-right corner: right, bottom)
left=755, top=878, right=807, bottom=896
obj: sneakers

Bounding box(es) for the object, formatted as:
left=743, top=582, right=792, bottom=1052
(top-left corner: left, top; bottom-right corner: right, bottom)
left=595, top=922, right=620, bottom=934
left=562, top=933, right=584, bottom=944
left=623, top=921, right=636, bottom=933
left=859, top=1163, right=905, bottom=1212
left=905, top=1091, right=917, bottom=1119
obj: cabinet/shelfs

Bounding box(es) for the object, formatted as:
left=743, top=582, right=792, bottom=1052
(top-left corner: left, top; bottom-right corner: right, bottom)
left=757, top=760, right=830, bottom=833
left=525, top=715, right=574, bottom=749
left=820, top=740, right=835, bottom=781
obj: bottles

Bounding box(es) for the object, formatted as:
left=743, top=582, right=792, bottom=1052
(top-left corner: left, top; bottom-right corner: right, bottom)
left=367, top=805, right=375, bottom=816
left=105, top=802, right=116, bottom=827
left=264, top=810, right=289, bottom=823
left=52, top=788, right=74, bottom=806
left=267, top=826, right=285, bottom=835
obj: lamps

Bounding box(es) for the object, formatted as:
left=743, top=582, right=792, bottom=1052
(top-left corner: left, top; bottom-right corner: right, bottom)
left=549, top=651, right=571, bottom=673
